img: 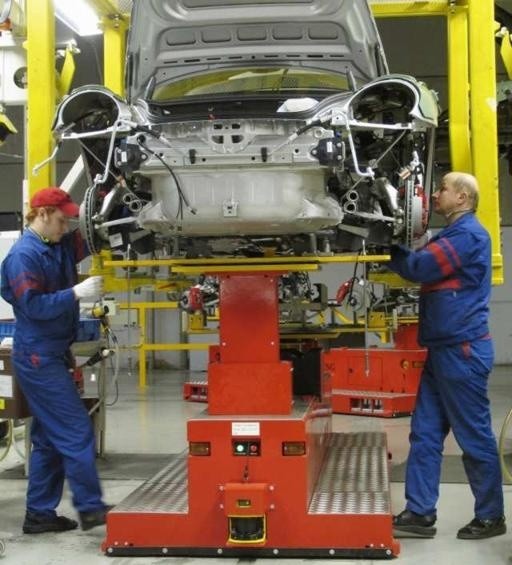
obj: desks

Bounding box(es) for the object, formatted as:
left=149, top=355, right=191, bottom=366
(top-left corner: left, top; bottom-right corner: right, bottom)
left=24, top=336, right=108, bottom=476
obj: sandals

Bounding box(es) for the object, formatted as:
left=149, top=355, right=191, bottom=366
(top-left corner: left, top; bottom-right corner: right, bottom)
left=458, top=516, right=506, bottom=538
left=394, top=510, right=436, bottom=535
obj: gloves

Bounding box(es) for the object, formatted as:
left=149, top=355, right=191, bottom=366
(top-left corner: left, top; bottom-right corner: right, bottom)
left=72, top=276, right=105, bottom=297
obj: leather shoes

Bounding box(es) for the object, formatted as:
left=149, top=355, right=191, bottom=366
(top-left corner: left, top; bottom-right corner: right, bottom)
left=80, top=506, right=115, bottom=530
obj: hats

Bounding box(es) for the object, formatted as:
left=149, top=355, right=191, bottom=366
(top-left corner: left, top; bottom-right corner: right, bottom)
left=31, top=188, right=80, bottom=217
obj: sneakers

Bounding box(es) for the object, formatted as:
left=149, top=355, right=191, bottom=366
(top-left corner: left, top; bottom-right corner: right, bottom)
left=23, top=510, right=78, bottom=533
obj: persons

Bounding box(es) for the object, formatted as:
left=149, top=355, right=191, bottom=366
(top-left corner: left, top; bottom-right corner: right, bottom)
left=0, top=188, right=116, bottom=534
left=377, top=170, right=507, bottom=540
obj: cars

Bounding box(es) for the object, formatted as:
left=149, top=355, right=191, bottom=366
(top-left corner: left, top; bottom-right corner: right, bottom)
left=31, top=1, right=442, bottom=255
